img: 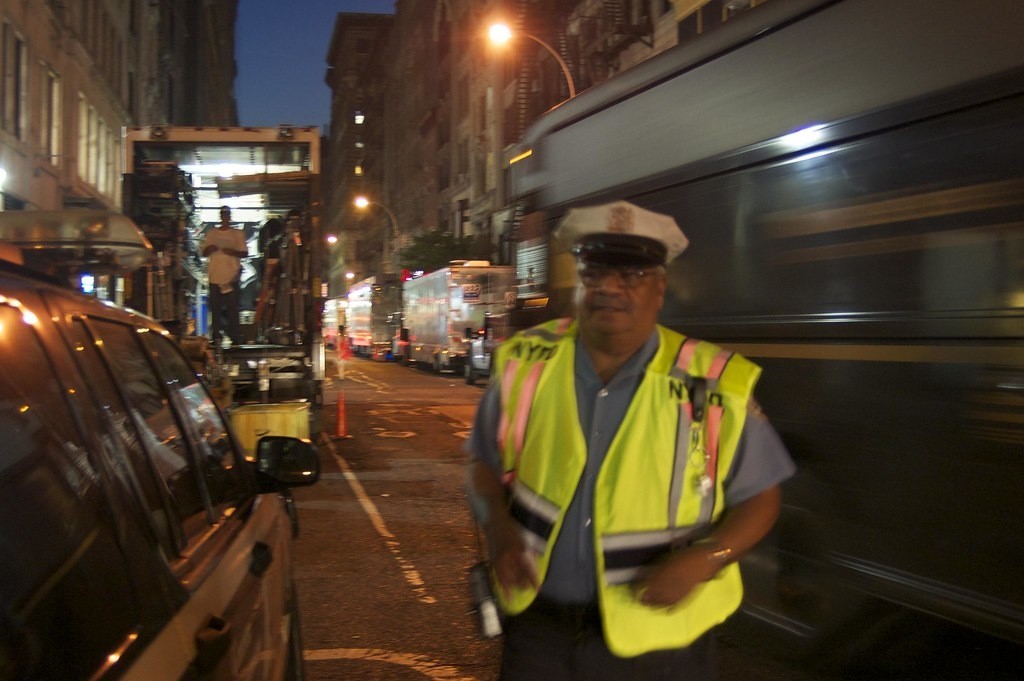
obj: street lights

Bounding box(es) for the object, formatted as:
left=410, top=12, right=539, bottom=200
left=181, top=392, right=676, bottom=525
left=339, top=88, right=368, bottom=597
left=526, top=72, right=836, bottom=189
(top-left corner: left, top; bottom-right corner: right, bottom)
left=354, top=196, right=401, bottom=277
left=488, top=22, right=575, bottom=107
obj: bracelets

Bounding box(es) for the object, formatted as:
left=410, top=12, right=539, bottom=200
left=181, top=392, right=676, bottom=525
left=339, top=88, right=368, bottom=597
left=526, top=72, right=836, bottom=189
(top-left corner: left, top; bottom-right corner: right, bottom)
left=693, top=536, right=731, bottom=580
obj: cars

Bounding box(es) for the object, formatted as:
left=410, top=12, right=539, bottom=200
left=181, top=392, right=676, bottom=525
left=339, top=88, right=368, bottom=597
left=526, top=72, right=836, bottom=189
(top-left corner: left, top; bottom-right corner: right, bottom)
left=462, top=327, right=499, bottom=386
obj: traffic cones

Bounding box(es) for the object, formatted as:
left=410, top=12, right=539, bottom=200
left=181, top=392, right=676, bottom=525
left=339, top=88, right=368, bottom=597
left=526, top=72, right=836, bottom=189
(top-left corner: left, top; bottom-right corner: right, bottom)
left=331, top=391, right=355, bottom=441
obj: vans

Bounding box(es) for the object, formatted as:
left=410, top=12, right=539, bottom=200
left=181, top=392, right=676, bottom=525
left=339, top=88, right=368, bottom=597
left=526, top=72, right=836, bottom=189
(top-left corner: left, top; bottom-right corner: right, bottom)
left=0, top=261, right=328, bottom=681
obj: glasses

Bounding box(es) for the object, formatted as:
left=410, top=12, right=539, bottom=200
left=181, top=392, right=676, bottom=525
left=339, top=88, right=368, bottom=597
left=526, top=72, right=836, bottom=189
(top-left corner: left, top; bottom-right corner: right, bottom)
left=575, top=267, right=660, bottom=288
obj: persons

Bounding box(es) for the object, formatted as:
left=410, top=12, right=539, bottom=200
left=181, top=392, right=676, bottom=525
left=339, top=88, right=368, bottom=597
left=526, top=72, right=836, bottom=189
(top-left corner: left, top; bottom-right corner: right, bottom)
left=201, top=206, right=248, bottom=345
left=466, top=199, right=797, bottom=681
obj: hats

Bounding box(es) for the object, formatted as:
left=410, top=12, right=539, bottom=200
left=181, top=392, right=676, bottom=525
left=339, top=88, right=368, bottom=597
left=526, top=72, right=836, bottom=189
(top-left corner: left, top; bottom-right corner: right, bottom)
left=554, top=201, right=690, bottom=268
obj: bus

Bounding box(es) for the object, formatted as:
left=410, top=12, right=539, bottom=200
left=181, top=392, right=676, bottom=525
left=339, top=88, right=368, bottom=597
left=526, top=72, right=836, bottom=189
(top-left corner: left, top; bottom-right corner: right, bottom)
left=321, top=297, right=348, bottom=349
left=345, top=274, right=408, bottom=362
left=402, top=259, right=519, bottom=375
left=499, top=0, right=1023, bottom=680
